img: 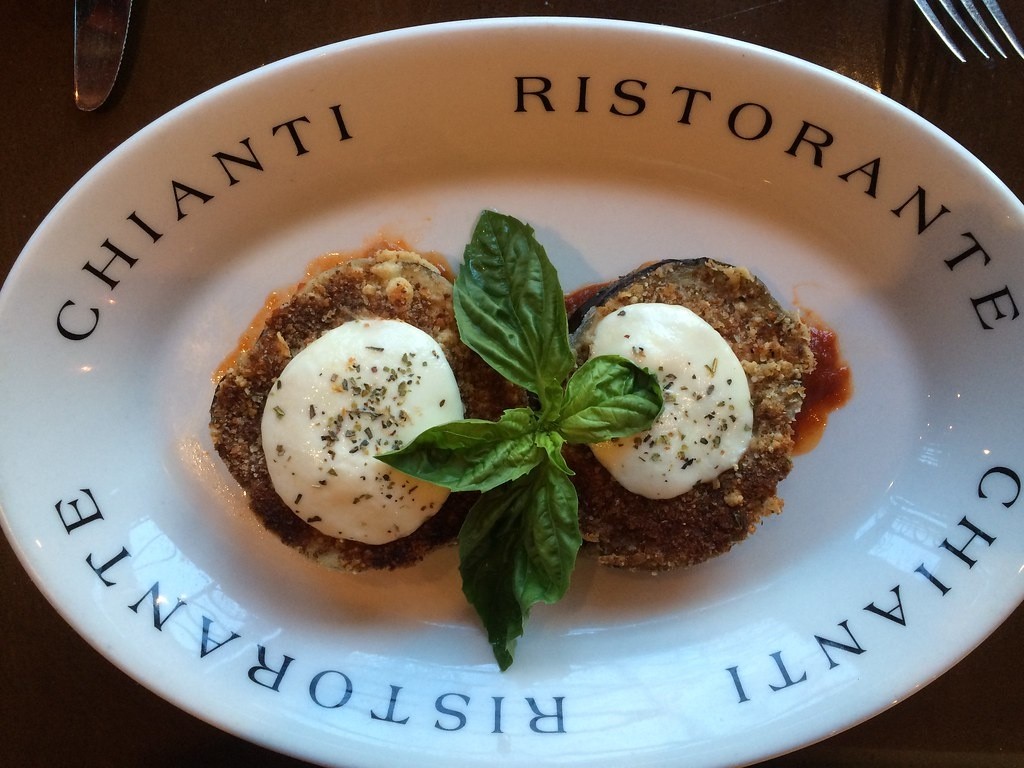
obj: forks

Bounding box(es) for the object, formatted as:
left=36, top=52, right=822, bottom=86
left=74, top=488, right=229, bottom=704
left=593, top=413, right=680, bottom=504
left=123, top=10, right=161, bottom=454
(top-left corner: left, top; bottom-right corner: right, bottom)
left=916, top=0, right=1024, bottom=63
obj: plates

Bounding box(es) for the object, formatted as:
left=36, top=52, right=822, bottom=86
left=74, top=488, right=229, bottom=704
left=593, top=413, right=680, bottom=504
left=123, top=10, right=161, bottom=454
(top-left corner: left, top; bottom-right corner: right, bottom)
left=0, top=15, right=1024, bottom=768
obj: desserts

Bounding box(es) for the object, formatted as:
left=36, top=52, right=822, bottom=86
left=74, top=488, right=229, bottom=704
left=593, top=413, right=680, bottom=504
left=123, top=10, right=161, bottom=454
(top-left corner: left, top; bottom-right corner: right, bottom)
left=208, top=249, right=530, bottom=573
left=532, top=258, right=819, bottom=574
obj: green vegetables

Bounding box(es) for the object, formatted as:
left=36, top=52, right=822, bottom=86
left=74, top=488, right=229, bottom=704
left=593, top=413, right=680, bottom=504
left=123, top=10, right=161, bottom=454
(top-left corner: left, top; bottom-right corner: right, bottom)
left=372, top=208, right=664, bottom=673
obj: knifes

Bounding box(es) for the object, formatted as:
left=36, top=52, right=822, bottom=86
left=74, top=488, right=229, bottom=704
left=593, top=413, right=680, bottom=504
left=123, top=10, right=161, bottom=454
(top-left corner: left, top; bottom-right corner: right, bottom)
left=73, top=0, right=134, bottom=111
